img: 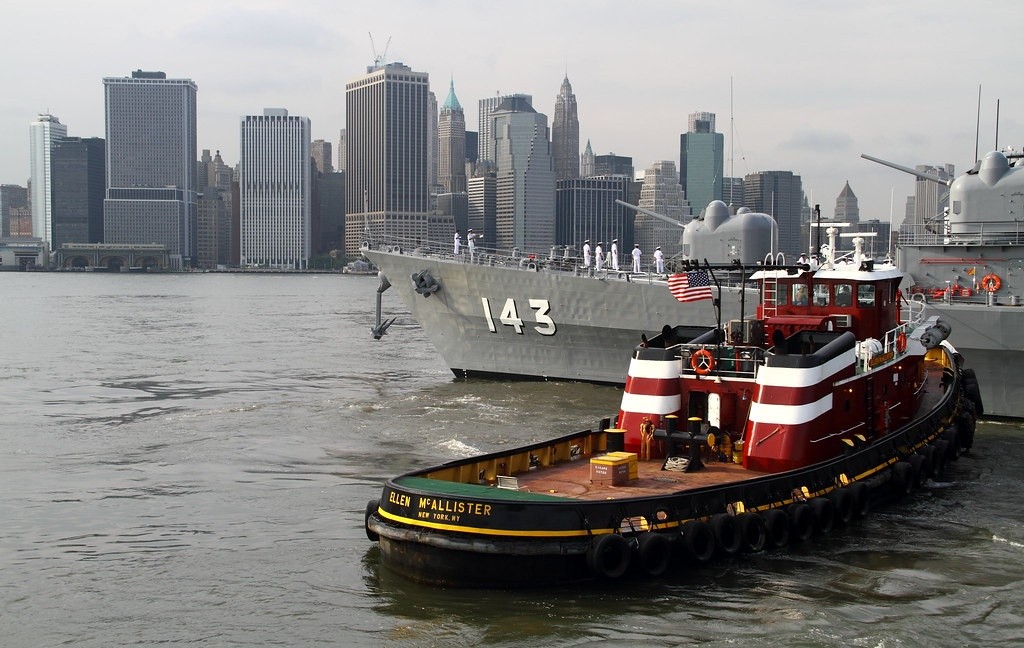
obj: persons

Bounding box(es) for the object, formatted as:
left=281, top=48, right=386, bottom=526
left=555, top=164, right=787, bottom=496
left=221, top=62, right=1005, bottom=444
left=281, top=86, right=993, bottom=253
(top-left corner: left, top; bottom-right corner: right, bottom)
left=798, top=253, right=806, bottom=290
left=840, top=255, right=853, bottom=294
left=596, top=241, right=604, bottom=274
left=468, top=229, right=481, bottom=255
left=836, top=287, right=860, bottom=306
left=796, top=287, right=806, bottom=301
left=612, top=239, right=620, bottom=269
left=454, top=230, right=462, bottom=255
left=632, top=244, right=642, bottom=273
left=654, top=246, right=664, bottom=274
left=583, top=240, right=591, bottom=266
left=811, top=255, right=820, bottom=266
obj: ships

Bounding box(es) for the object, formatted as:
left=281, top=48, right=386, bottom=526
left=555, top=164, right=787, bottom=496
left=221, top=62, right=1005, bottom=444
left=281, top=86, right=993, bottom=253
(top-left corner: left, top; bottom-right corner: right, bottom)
left=358, top=75, right=1024, bottom=418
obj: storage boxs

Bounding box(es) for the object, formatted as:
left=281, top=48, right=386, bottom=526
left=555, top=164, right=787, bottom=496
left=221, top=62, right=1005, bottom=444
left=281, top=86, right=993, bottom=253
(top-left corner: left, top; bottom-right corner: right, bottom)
left=607, top=452, right=639, bottom=481
left=590, top=456, right=629, bottom=487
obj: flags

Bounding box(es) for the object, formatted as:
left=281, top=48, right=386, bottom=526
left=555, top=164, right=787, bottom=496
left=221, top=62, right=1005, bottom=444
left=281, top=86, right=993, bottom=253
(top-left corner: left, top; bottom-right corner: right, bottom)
left=968, top=269, right=974, bottom=275
left=668, top=270, right=713, bottom=303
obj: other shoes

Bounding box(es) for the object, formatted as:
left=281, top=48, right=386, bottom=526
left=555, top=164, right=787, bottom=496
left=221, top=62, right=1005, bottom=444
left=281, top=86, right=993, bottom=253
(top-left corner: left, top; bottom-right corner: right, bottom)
left=661, top=272, right=665, bottom=274
left=657, top=273, right=659, bottom=275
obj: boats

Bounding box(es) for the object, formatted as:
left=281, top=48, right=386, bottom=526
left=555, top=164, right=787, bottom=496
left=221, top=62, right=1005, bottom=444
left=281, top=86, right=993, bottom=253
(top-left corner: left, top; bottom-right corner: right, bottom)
left=365, top=227, right=985, bottom=589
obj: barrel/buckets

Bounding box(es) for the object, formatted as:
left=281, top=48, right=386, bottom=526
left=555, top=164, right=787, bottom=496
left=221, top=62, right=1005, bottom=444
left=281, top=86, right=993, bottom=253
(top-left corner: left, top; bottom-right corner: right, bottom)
left=921, top=328, right=943, bottom=348
left=933, top=321, right=951, bottom=340
left=856, top=339, right=882, bottom=360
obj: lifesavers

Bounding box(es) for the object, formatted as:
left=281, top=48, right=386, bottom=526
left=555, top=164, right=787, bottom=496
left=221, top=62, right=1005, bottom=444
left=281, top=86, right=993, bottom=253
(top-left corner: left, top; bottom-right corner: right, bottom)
left=675, top=520, right=717, bottom=564
left=588, top=532, right=634, bottom=582
left=760, top=508, right=790, bottom=548
left=629, top=531, right=672, bottom=580
left=691, top=349, right=715, bottom=375
left=982, top=274, right=1001, bottom=291
left=735, top=512, right=767, bottom=552
left=364, top=498, right=380, bottom=542
left=786, top=504, right=814, bottom=540
left=810, top=369, right=985, bottom=535
left=898, top=331, right=906, bottom=353
left=708, top=513, right=742, bottom=554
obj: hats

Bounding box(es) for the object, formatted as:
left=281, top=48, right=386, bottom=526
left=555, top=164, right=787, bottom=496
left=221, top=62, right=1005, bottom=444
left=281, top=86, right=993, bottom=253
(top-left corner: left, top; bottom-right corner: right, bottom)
left=656, top=247, right=660, bottom=250
left=584, top=240, right=590, bottom=243
left=468, top=229, right=472, bottom=232
left=612, top=239, right=617, bottom=243
left=634, top=244, right=639, bottom=247
left=597, top=242, right=603, bottom=245
